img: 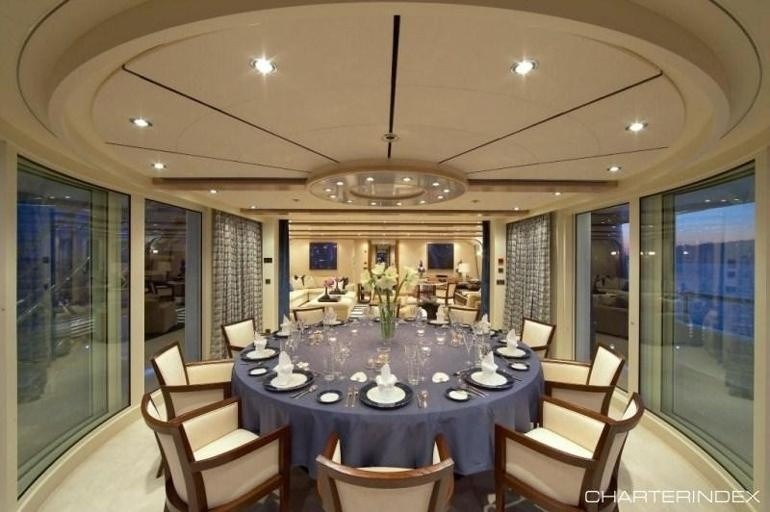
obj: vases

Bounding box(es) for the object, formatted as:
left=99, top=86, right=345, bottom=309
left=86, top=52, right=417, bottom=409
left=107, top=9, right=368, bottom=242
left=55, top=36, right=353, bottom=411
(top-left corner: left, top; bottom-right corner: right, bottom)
left=318, top=286, right=331, bottom=301
left=380, top=305, right=395, bottom=337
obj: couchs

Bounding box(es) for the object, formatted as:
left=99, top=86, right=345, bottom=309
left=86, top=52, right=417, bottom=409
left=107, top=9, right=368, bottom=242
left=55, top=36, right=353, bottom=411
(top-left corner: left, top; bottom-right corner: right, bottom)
left=289, top=290, right=309, bottom=309
left=594, top=294, right=691, bottom=344
left=145, top=295, right=178, bottom=334
left=426, top=275, right=454, bottom=288
left=455, top=278, right=484, bottom=309
left=293, top=276, right=356, bottom=323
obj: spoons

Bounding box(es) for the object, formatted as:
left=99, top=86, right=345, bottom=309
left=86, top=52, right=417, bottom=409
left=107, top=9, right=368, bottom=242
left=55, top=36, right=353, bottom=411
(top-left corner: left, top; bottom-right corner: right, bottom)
left=294, top=384, right=318, bottom=400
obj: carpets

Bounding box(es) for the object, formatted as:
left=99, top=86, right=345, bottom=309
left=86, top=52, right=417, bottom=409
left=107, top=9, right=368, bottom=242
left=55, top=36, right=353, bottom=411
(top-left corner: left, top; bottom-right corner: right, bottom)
left=347, top=304, right=427, bottom=322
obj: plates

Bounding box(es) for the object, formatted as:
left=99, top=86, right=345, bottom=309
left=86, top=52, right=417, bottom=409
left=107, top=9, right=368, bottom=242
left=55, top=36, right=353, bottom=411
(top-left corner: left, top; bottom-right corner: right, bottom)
left=273, top=331, right=290, bottom=339
left=498, top=337, right=507, bottom=344
left=240, top=347, right=280, bottom=362
left=320, top=319, right=344, bottom=327
left=358, top=383, right=414, bottom=409
left=263, top=370, right=317, bottom=392
left=508, top=362, right=530, bottom=372
left=316, top=390, right=344, bottom=403
left=489, top=329, right=498, bottom=337
left=247, top=366, right=270, bottom=376
left=427, top=319, right=450, bottom=325
left=493, top=344, right=533, bottom=360
left=462, top=368, right=515, bottom=390
left=443, top=387, right=472, bottom=402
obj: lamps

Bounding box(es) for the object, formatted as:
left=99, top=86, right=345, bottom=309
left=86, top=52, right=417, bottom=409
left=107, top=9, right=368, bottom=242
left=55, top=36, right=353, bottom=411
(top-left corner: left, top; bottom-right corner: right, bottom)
left=458, top=263, right=470, bottom=282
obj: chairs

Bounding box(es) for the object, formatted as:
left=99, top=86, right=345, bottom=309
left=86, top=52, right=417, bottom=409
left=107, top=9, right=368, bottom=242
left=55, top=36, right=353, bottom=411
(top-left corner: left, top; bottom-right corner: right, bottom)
left=436, top=283, right=457, bottom=305
left=149, top=340, right=235, bottom=420
left=494, top=393, right=645, bottom=512
left=222, top=318, right=255, bottom=364
left=448, top=307, right=479, bottom=323
left=540, top=344, right=625, bottom=416
left=315, top=432, right=454, bottom=512
left=521, top=318, right=555, bottom=358
left=370, top=305, right=400, bottom=320
left=293, top=306, right=324, bottom=322
left=140, top=393, right=293, bottom=512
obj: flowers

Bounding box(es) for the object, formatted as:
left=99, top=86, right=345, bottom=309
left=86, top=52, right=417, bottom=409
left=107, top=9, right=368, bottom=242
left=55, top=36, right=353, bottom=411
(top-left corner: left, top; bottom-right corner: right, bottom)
left=360, top=263, right=418, bottom=339
left=322, top=278, right=334, bottom=295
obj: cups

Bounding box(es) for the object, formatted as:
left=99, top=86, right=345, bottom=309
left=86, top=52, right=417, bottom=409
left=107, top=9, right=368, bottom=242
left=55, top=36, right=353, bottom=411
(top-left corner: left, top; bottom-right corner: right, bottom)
left=433, top=327, right=448, bottom=346
left=461, top=333, right=474, bottom=366
left=349, top=322, right=359, bottom=337
left=414, top=320, right=427, bottom=336
left=327, top=333, right=338, bottom=354
left=362, top=351, right=377, bottom=369
left=322, top=346, right=335, bottom=381
left=290, top=329, right=302, bottom=348
left=376, top=347, right=391, bottom=370
left=417, top=339, right=432, bottom=382
left=408, top=358, right=420, bottom=386
left=280, top=338, right=292, bottom=360
left=405, top=344, right=417, bottom=366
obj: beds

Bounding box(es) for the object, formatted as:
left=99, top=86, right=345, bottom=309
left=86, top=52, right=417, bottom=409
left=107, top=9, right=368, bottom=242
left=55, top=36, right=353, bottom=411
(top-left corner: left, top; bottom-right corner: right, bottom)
left=231, top=321, right=545, bottom=479
